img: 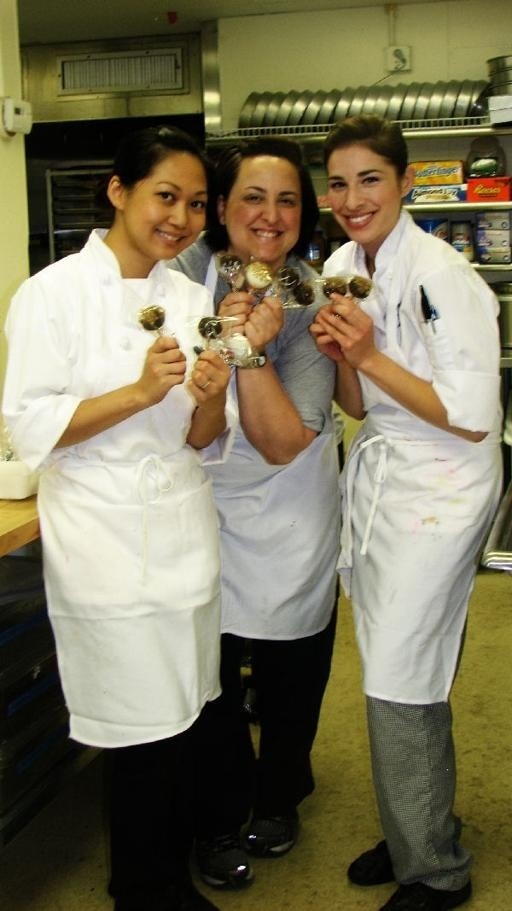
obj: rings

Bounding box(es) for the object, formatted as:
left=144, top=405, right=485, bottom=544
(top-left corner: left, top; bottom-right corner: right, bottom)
left=202, top=382, right=210, bottom=389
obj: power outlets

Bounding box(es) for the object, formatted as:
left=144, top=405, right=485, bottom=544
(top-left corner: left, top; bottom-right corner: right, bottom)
left=385, top=45, right=411, bottom=73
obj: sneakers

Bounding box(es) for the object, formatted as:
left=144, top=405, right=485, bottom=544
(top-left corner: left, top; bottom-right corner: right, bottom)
left=195, top=834, right=254, bottom=887
left=248, top=818, right=297, bottom=854
left=379, top=882, right=471, bottom=911
left=347, top=839, right=395, bottom=886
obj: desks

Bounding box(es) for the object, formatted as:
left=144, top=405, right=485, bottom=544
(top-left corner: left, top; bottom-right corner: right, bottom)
left=0, top=492, right=110, bottom=854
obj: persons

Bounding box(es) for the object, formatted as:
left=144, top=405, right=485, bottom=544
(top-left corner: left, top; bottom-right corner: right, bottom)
left=305, top=114, right=506, bottom=911
left=163, top=136, right=346, bottom=894
left=1, top=126, right=257, bottom=911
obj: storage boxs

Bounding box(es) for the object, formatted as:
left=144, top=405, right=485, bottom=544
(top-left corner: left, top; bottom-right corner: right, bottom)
left=408, top=157, right=466, bottom=185
left=473, top=213, right=512, bottom=267
left=466, top=176, right=511, bottom=202
left=407, top=185, right=467, bottom=205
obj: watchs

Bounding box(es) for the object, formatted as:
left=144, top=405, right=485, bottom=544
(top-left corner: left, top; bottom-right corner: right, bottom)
left=238, top=352, right=267, bottom=368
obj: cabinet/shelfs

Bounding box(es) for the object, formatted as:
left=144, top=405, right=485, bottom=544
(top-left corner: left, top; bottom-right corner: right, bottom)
left=209, top=117, right=511, bottom=454
left=45, top=156, right=118, bottom=266
